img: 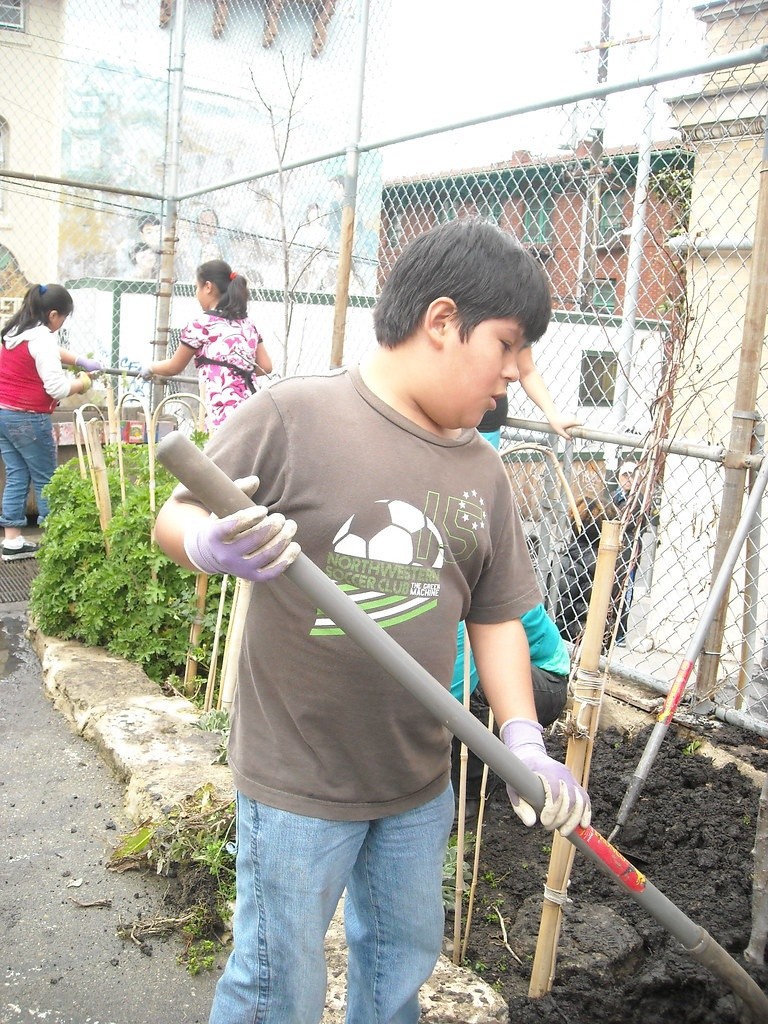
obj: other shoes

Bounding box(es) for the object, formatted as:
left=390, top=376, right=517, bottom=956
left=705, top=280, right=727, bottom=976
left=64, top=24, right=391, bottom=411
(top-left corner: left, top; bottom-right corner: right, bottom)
left=452, top=767, right=499, bottom=829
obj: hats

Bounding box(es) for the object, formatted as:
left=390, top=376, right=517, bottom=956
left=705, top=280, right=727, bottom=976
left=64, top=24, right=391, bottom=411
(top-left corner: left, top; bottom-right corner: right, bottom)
left=619, top=462, right=637, bottom=477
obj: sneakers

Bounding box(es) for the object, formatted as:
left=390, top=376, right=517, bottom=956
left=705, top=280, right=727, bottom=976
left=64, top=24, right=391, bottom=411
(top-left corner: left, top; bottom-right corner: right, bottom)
left=1, top=541, right=44, bottom=561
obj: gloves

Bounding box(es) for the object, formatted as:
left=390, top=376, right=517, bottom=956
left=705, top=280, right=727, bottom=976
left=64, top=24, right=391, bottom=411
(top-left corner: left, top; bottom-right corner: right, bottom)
left=137, top=363, right=153, bottom=381
left=499, top=718, right=591, bottom=837
left=77, top=371, right=92, bottom=394
left=183, top=475, right=301, bottom=582
left=75, top=356, right=102, bottom=372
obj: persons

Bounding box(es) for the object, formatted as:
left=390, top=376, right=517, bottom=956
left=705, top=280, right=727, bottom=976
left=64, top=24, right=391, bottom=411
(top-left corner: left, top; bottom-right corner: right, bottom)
left=542, top=461, right=651, bottom=656
left=140, top=259, right=272, bottom=440
left=451, top=602, right=570, bottom=830
left=0, top=284, right=107, bottom=561
left=477, top=344, right=587, bottom=451
left=154, top=219, right=592, bottom=1024
